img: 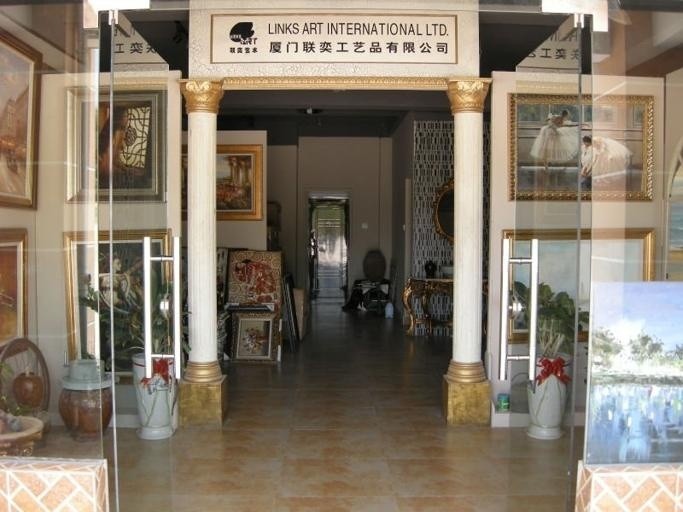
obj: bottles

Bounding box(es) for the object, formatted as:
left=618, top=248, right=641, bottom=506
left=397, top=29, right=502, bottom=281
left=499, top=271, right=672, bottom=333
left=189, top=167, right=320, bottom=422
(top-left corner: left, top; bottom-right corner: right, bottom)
left=385, top=300, right=393, bottom=318
left=497, top=394, right=510, bottom=413
left=425, top=260, right=437, bottom=278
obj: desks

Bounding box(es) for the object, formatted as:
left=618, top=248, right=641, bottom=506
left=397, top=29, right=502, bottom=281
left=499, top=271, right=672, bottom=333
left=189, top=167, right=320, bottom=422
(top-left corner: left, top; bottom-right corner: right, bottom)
left=359, top=279, right=390, bottom=315
left=402, top=277, right=487, bottom=344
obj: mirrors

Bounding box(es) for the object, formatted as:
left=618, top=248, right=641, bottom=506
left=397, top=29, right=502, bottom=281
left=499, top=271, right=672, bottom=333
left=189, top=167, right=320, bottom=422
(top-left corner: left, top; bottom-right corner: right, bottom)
left=431, top=176, right=454, bottom=242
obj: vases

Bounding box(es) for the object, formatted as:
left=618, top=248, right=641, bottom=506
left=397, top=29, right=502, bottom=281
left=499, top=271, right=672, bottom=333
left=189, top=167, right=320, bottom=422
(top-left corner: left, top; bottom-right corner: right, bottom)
left=58, top=358, right=119, bottom=439
left=12, top=372, right=43, bottom=414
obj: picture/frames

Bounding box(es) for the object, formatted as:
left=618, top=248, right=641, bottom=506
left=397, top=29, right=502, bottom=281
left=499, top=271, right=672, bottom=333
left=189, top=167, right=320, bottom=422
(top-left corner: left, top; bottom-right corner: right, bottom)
left=216, top=246, right=300, bottom=366
left=0, top=27, right=43, bottom=211
left=64, top=81, right=170, bottom=206
left=59, top=227, right=174, bottom=385
left=502, top=226, right=657, bottom=343
left=505, top=92, right=656, bottom=202
left=181, top=141, right=266, bottom=222
left=0, top=227, right=29, bottom=359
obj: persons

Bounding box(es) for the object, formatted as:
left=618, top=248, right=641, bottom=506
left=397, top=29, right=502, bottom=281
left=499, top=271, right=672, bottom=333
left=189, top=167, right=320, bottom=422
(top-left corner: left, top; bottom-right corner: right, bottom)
left=97, top=251, right=143, bottom=350
left=529, top=106, right=590, bottom=174
left=97, top=104, right=148, bottom=184
left=577, top=129, right=634, bottom=187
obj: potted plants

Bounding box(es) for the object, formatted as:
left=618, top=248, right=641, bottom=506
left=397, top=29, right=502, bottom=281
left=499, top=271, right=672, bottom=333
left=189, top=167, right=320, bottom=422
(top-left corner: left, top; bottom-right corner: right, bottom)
left=77, top=264, right=192, bottom=440
left=0, top=363, right=45, bottom=457
left=513, top=280, right=589, bottom=441
